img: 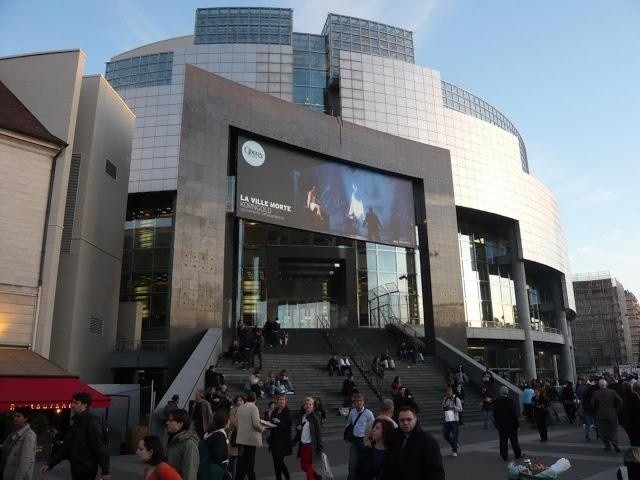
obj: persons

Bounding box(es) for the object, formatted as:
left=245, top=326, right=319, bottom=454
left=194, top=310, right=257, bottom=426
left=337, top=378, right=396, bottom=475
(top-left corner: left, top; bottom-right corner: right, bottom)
left=372, top=342, right=425, bottom=380
left=167, top=411, right=199, bottom=480
left=292, top=397, right=331, bottom=480
left=327, top=354, right=360, bottom=396
left=480, top=367, right=640, bottom=461
left=306, top=181, right=400, bottom=245
left=366, top=418, right=395, bottom=476
left=0, top=443, right=2, bottom=443
left=219, top=318, right=289, bottom=371
left=391, top=376, right=419, bottom=419
left=41, top=393, right=111, bottom=480
left=267, top=395, right=294, bottom=480
left=441, top=362, right=468, bottom=457
left=395, top=405, right=445, bottom=478
left=235, top=391, right=265, bottom=479
left=166, top=366, right=296, bottom=435
left=343, top=393, right=375, bottom=479
left=617, top=447, right=639, bottom=479
left=136, top=435, right=182, bottom=480
left=202, top=412, right=231, bottom=479
left=2, top=408, right=35, bottom=480
left=313, top=399, right=327, bottom=424
left=227, top=409, right=242, bottom=480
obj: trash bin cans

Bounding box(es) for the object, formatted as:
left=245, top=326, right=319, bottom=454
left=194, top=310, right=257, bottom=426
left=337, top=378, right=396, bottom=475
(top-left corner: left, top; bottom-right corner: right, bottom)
left=508, top=471, right=558, bottom=480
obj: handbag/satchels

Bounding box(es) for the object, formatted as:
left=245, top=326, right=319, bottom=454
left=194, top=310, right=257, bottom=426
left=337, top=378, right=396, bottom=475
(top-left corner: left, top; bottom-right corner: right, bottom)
left=290, top=425, right=303, bottom=447
left=344, top=424, right=354, bottom=442
left=444, top=410, right=455, bottom=422
left=320, top=452, right=334, bottom=479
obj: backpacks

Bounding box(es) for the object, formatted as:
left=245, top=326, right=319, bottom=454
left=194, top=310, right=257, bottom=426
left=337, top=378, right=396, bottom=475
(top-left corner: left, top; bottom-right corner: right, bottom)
left=454, top=396, right=463, bottom=425
